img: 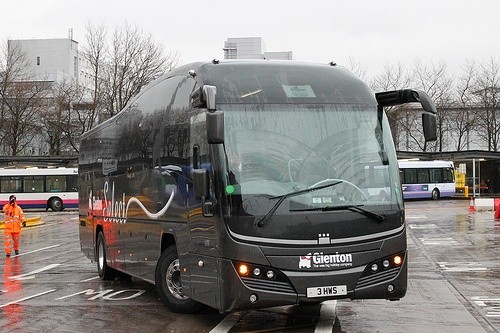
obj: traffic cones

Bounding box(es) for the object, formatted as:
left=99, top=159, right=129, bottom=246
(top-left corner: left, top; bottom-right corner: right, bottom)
left=468, top=196, right=476, bottom=212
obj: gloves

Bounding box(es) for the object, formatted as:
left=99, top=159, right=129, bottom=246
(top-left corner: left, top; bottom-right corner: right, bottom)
left=10, top=199, right=14, bottom=206
left=22, top=222, right=26, bottom=227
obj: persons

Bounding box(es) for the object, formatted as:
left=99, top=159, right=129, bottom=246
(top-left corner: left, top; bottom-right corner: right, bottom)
left=2, top=195, right=26, bottom=258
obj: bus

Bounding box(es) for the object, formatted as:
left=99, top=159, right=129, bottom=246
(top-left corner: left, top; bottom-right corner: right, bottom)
left=0, top=165, right=79, bottom=211
left=397, top=159, right=456, bottom=200
left=77, top=58, right=437, bottom=312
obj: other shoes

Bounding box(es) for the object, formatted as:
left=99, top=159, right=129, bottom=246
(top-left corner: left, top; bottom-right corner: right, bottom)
left=7, top=253, right=10, bottom=256
left=15, top=250, right=19, bottom=255
